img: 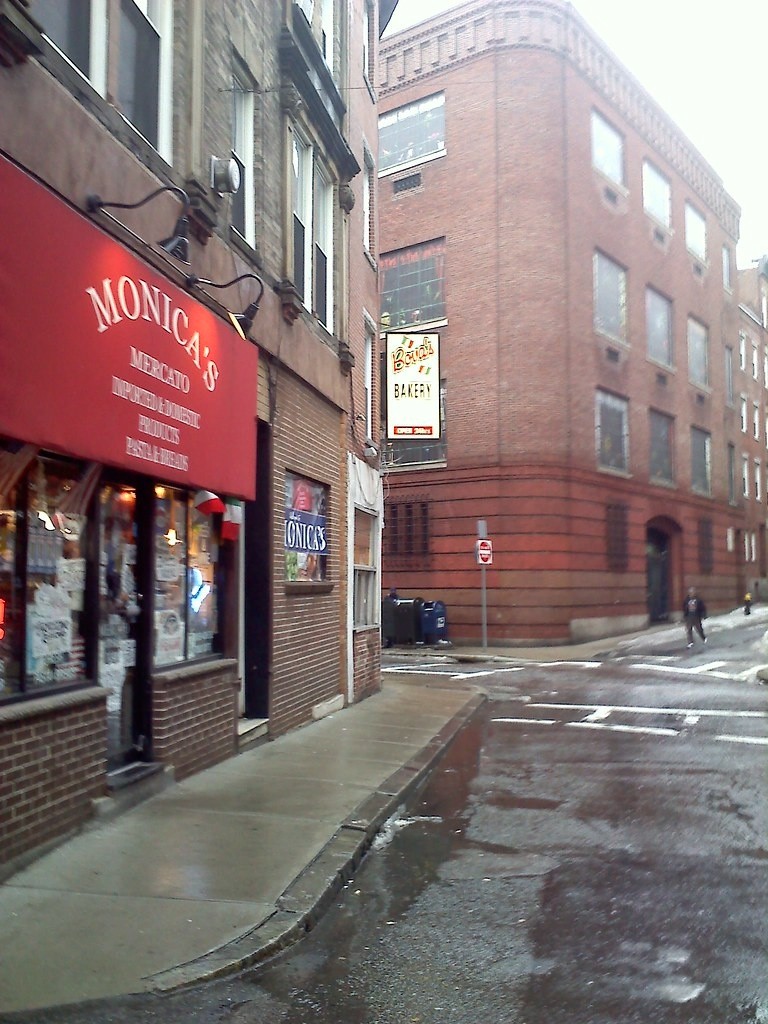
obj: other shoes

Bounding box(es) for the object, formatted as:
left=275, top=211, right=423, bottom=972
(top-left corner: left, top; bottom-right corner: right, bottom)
left=687, top=642, right=693, bottom=648
left=704, top=638, right=707, bottom=643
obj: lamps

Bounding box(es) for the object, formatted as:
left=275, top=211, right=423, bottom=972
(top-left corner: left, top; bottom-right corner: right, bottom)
left=88, top=186, right=192, bottom=266
left=187, top=274, right=264, bottom=340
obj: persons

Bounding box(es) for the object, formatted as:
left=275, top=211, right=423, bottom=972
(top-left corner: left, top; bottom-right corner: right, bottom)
left=684, top=587, right=707, bottom=648
left=745, top=591, right=753, bottom=616
